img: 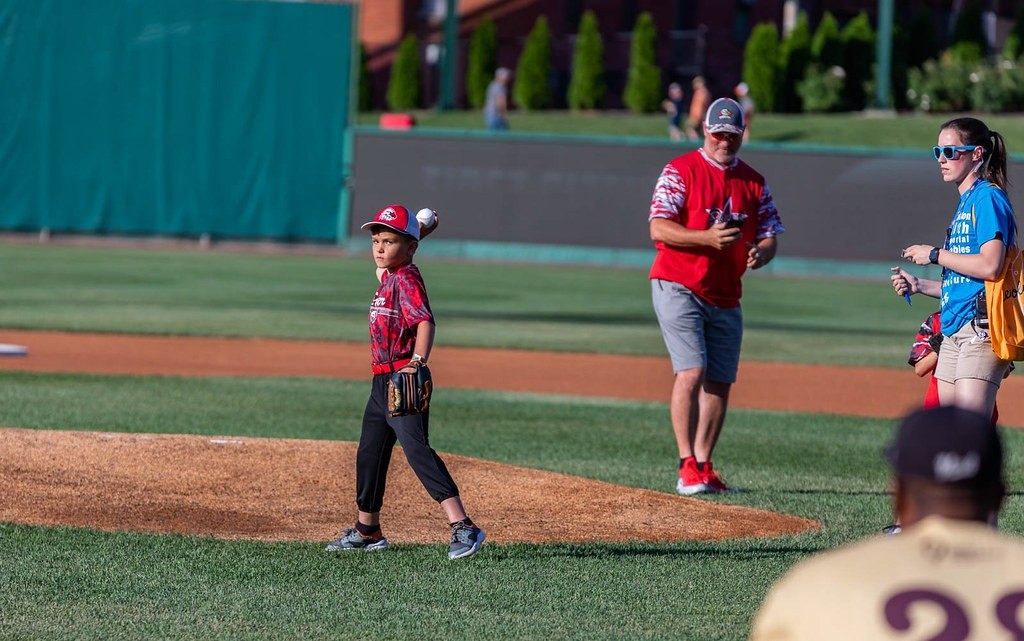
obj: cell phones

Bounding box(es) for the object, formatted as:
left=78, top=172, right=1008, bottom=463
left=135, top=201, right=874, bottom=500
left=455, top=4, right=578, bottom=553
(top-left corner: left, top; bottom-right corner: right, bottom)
left=723, top=220, right=744, bottom=237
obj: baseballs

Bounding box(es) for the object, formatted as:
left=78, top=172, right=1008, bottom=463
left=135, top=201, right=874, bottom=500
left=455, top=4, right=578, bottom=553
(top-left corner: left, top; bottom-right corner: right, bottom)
left=416, top=208, right=435, bottom=229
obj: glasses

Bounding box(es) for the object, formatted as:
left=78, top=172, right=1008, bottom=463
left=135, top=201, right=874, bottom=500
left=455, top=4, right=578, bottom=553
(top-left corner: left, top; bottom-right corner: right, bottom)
left=711, top=132, right=741, bottom=142
left=933, top=146, right=986, bottom=161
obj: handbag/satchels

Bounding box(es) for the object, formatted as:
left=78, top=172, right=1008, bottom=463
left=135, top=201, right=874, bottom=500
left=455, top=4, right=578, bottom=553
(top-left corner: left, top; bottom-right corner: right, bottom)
left=971, top=181, right=1024, bottom=362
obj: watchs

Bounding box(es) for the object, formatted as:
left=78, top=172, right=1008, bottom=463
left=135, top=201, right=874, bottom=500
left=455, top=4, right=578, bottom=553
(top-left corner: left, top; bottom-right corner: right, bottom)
left=929, top=248, right=941, bottom=264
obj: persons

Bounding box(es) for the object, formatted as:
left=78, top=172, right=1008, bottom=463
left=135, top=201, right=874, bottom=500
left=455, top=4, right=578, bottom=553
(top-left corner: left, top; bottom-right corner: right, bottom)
left=746, top=408, right=1023, bottom=640
left=326, top=205, right=487, bottom=558
left=876, top=115, right=1024, bottom=534
left=661, top=77, right=758, bottom=144
left=484, top=68, right=513, bottom=131
left=648, top=96, right=784, bottom=493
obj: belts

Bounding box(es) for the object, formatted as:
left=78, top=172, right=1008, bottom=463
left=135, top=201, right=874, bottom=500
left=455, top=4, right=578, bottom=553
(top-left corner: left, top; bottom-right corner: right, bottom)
left=371, top=358, right=410, bottom=375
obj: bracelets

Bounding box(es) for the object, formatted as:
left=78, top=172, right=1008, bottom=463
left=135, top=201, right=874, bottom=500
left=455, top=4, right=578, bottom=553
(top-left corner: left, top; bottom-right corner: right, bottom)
left=411, top=354, right=425, bottom=363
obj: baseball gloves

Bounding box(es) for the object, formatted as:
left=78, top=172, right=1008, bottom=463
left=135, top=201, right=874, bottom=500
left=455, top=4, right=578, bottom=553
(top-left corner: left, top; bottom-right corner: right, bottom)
left=929, top=332, right=946, bottom=355
left=385, top=354, right=434, bottom=418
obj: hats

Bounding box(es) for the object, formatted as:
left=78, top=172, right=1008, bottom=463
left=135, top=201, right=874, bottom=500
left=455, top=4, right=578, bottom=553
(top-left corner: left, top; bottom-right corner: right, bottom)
left=360, top=203, right=420, bottom=240
left=883, top=404, right=1004, bottom=487
left=705, top=98, right=745, bottom=135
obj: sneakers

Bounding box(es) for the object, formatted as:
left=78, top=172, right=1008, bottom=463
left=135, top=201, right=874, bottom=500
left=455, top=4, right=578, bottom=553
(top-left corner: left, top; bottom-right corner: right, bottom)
left=325, top=528, right=389, bottom=552
left=676, top=457, right=709, bottom=496
left=446, top=521, right=486, bottom=560
left=701, top=461, right=738, bottom=495
